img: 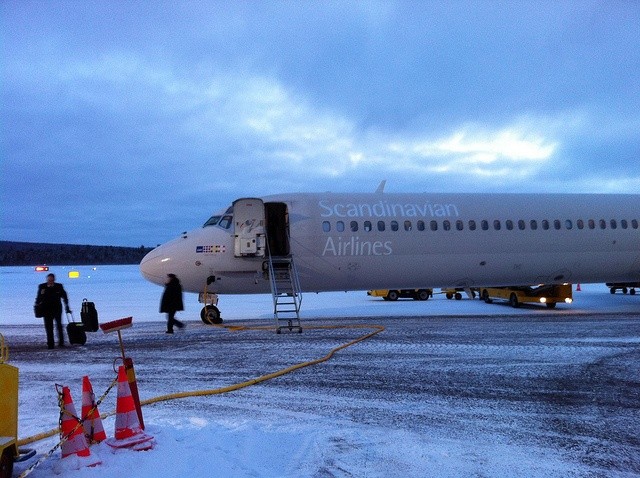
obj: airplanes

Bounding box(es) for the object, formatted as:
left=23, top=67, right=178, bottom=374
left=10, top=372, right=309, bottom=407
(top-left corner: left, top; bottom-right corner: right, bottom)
left=139, top=180, right=640, bottom=324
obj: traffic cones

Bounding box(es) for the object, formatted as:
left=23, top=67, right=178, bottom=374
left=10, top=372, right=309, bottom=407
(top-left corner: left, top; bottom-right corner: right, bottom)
left=576, top=283, right=580, bottom=290
left=53, top=386, right=102, bottom=474
left=105, top=364, right=153, bottom=447
left=80, top=376, right=106, bottom=447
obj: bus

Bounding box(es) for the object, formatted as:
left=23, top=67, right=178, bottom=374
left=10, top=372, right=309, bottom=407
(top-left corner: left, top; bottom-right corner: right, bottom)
left=440, top=286, right=480, bottom=298
left=367, top=288, right=433, bottom=299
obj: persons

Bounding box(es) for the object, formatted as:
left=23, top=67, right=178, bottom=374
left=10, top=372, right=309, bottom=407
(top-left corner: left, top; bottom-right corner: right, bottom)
left=33, top=273, right=71, bottom=349
left=159, top=273, right=185, bottom=334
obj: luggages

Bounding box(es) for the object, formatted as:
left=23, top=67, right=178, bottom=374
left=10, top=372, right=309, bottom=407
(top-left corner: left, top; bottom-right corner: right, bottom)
left=66, top=309, right=86, bottom=346
left=80, top=297, right=99, bottom=331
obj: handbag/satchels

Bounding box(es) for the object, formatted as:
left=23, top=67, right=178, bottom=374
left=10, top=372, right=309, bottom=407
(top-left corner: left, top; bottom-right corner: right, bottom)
left=34, top=284, right=58, bottom=317
